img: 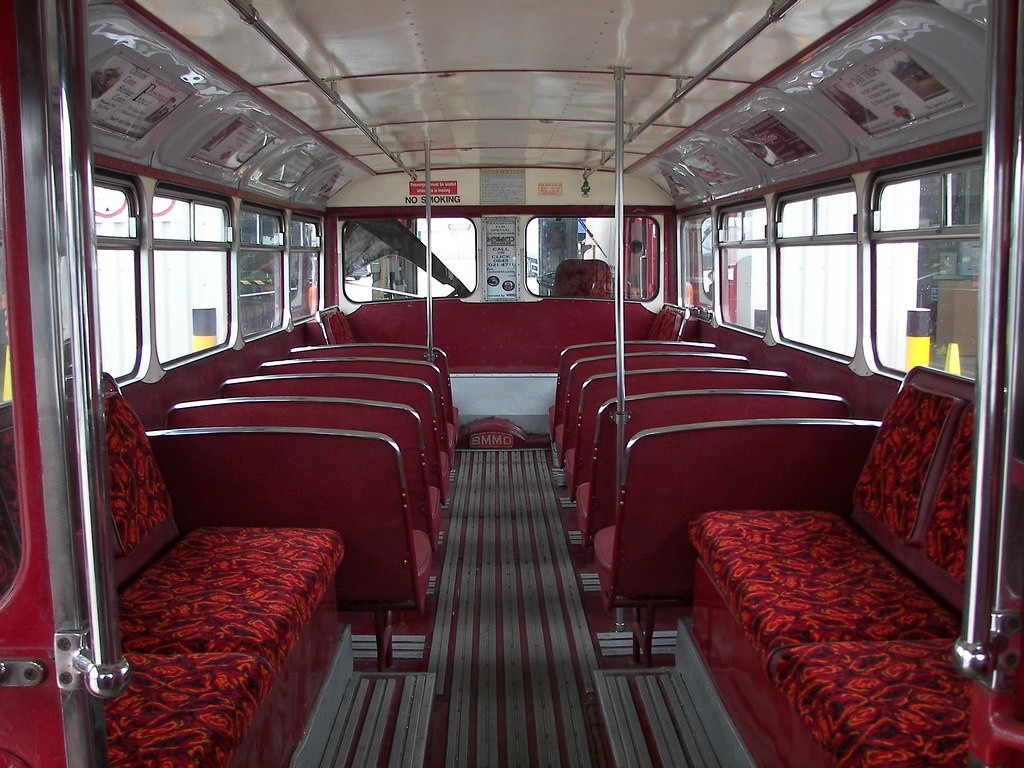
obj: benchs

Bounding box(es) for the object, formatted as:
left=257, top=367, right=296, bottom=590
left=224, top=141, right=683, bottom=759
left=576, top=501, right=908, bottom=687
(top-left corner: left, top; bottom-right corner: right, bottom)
left=549, top=301, right=1024, bottom=768
left=1, top=306, right=461, bottom=768
